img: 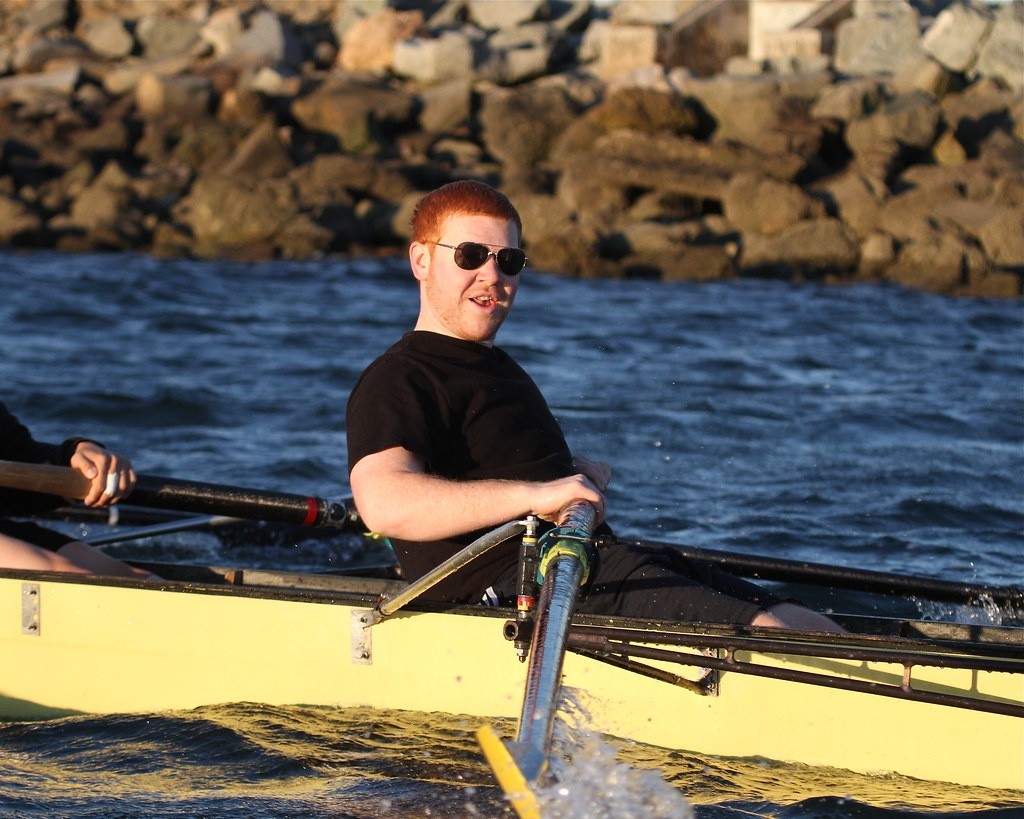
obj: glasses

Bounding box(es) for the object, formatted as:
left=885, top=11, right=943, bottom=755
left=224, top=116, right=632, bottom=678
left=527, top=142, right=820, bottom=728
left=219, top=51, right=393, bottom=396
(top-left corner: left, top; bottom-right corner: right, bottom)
left=428, top=241, right=529, bottom=273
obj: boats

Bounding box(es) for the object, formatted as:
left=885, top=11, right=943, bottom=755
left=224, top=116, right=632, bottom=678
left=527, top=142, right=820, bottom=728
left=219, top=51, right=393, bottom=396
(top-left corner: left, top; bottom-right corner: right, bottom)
left=0, top=560, right=1023, bottom=790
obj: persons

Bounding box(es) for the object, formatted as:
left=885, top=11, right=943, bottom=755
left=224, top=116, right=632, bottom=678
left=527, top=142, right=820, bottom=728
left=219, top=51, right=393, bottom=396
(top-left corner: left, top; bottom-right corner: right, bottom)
left=347, top=180, right=847, bottom=632
left=0, top=400, right=166, bottom=581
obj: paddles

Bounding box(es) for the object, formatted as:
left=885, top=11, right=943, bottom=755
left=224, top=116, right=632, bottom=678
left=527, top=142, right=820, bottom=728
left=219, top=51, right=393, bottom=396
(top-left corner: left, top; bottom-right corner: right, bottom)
left=0, top=461, right=1022, bottom=612
left=474, top=455, right=615, bottom=817
